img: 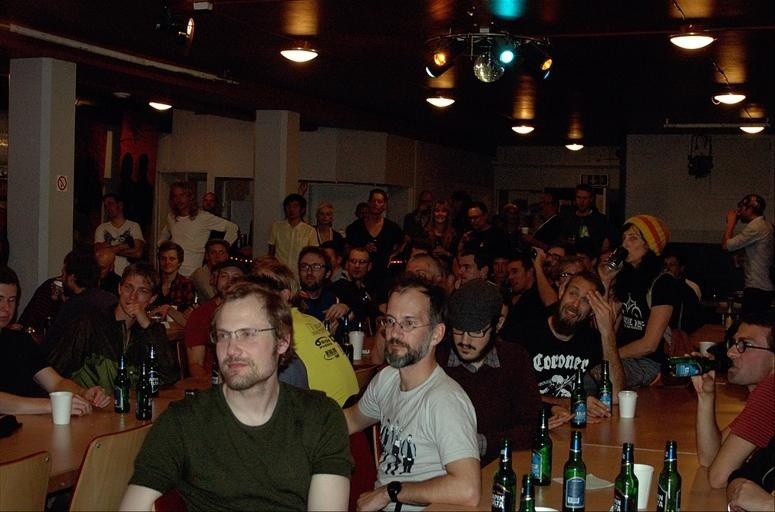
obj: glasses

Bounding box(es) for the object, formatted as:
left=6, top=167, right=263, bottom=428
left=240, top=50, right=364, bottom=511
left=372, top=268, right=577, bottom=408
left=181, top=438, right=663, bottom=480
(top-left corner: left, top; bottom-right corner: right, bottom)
left=380, top=317, right=433, bottom=331
left=298, top=263, right=330, bottom=272
left=729, top=340, right=770, bottom=355
left=210, top=327, right=275, bottom=343
left=453, top=326, right=497, bottom=338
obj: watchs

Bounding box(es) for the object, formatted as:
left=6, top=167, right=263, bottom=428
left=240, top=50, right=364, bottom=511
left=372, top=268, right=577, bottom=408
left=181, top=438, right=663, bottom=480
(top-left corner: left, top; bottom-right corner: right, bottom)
left=387, top=480, right=402, bottom=504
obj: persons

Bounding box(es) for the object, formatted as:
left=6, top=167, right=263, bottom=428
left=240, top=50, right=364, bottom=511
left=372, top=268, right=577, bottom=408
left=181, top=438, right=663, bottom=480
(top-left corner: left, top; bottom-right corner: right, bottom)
left=691, top=308, right=774, bottom=491
left=719, top=193, right=773, bottom=325
left=335, top=275, right=484, bottom=510
left=15, top=181, right=705, bottom=470
left=0, top=263, right=114, bottom=419
left=112, top=285, right=354, bottom=512
left=726, top=419, right=774, bottom=512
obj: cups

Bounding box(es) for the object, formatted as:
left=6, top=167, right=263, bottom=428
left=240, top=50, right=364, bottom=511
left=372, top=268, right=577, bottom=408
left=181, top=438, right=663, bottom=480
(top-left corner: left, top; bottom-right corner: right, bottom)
left=617, top=390, right=638, bottom=418
left=51, top=280, right=63, bottom=300
left=521, top=227, right=528, bottom=235
left=48, top=391, right=73, bottom=425
left=699, top=341, right=717, bottom=353
left=165, top=305, right=178, bottom=322
left=348, top=330, right=365, bottom=360
left=632, top=463, right=655, bottom=510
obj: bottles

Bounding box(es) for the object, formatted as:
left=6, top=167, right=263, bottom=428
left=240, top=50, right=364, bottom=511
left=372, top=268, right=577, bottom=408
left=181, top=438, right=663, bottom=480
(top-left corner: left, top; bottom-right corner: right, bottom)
left=570, top=369, right=588, bottom=428
left=614, top=440, right=640, bottom=512
left=340, top=317, right=348, bottom=345
left=323, top=319, right=330, bottom=332
left=656, top=439, right=682, bottom=512
left=598, top=359, right=613, bottom=417
left=147, top=344, right=160, bottom=397
left=562, top=430, right=588, bottom=512
left=519, top=474, right=537, bottom=512
left=531, top=248, right=553, bottom=268
left=135, top=360, right=153, bottom=420
left=724, top=296, right=737, bottom=336
left=490, top=440, right=517, bottom=512
left=113, top=355, right=131, bottom=413
left=532, top=408, right=553, bottom=486
left=666, top=356, right=732, bottom=379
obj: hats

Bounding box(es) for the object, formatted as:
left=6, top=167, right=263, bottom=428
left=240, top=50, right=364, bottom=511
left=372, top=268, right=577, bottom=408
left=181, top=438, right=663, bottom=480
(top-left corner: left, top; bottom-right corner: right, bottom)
left=737, top=194, right=767, bottom=209
left=624, top=215, right=671, bottom=257
